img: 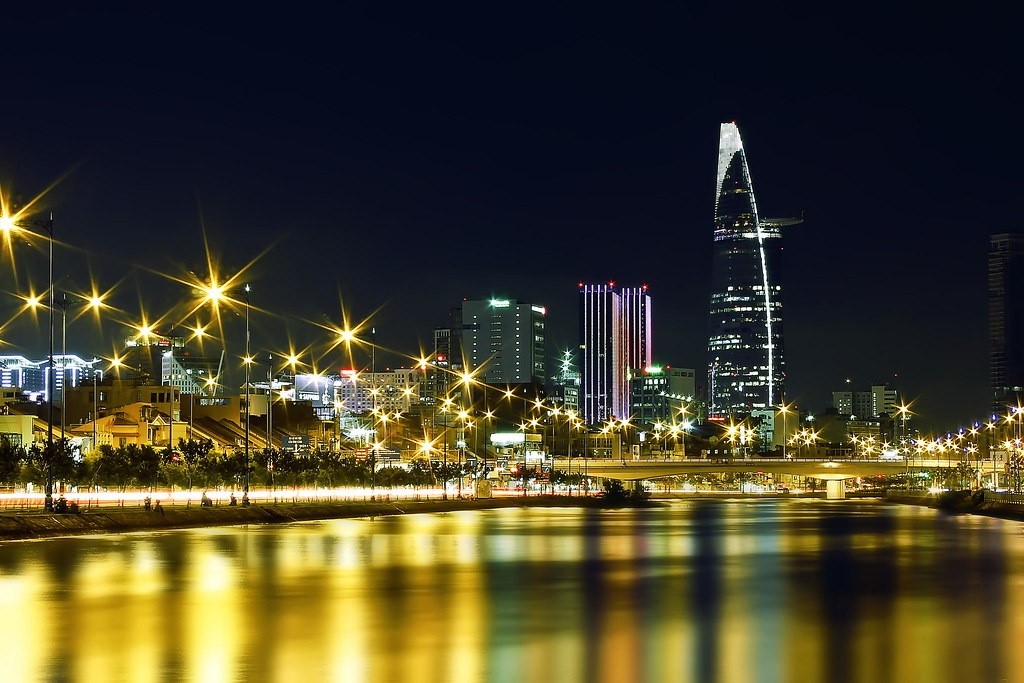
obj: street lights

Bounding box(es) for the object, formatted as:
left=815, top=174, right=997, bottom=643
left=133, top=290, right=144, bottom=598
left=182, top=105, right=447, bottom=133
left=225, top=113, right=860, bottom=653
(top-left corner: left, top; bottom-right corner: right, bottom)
left=460, top=374, right=488, bottom=481
left=19, top=290, right=102, bottom=500
left=242, top=350, right=297, bottom=473
left=208, top=283, right=250, bottom=508
left=901, top=406, right=908, bottom=460
left=519, top=424, right=527, bottom=468
left=416, top=359, right=449, bottom=499
left=139, top=322, right=206, bottom=453
left=781, top=407, right=788, bottom=459
left=342, top=331, right=376, bottom=491
left=535, top=400, right=555, bottom=471
left=0, top=218, right=55, bottom=509
